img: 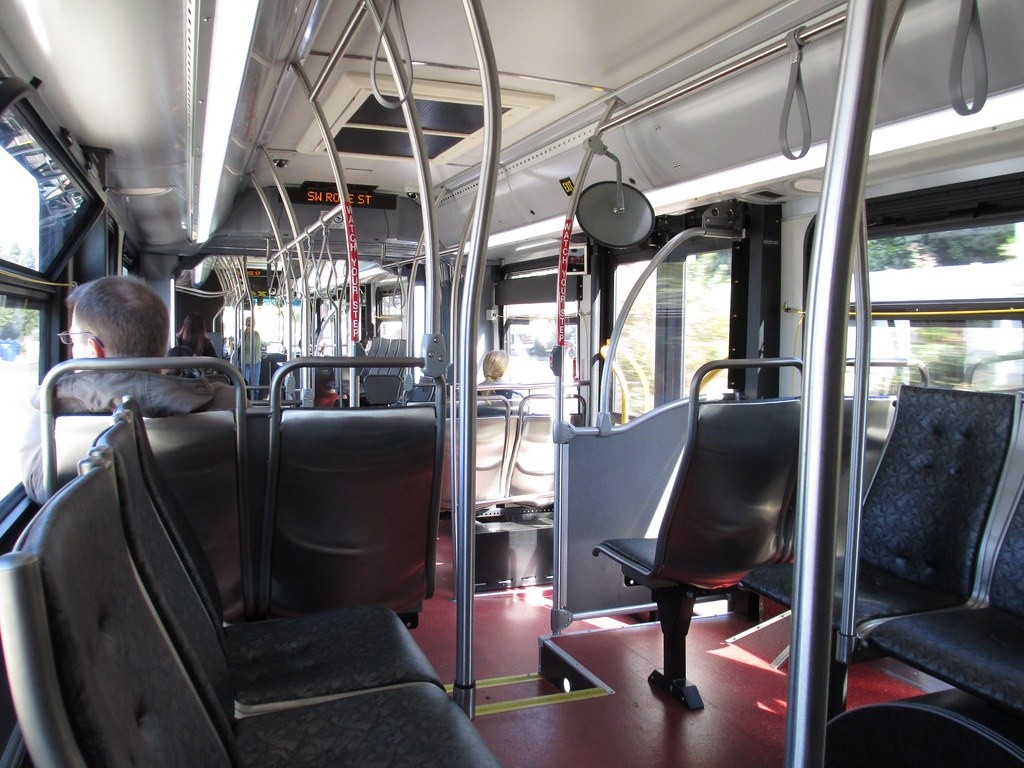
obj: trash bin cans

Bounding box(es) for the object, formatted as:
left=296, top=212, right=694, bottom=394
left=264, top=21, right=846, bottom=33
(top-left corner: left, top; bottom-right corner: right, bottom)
left=0, top=339, right=19, bottom=361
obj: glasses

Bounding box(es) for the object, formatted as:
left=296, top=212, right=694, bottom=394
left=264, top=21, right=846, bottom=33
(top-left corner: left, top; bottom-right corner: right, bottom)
left=57, top=330, right=104, bottom=348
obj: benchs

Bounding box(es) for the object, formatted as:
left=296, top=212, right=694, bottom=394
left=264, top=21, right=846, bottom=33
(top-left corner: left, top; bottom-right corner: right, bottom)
left=0, top=336, right=1024, bottom=768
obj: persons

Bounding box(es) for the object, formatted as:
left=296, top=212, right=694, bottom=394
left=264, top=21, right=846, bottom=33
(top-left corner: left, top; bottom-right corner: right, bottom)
left=162, top=346, right=205, bottom=378
left=174, top=313, right=218, bottom=358
left=19, top=275, right=251, bottom=503
left=231, top=317, right=261, bottom=402
left=474, top=349, right=514, bottom=406
